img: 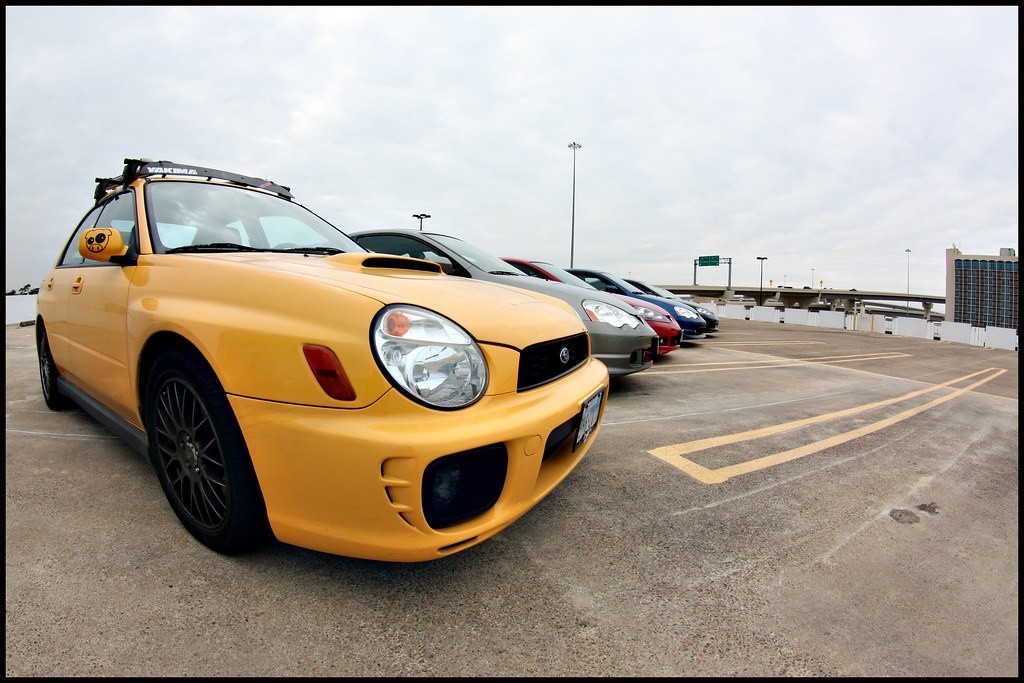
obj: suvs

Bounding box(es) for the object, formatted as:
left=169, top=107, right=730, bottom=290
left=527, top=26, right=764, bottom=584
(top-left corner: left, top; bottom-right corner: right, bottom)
left=804, top=287, right=810, bottom=289
left=35, top=157, right=609, bottom=562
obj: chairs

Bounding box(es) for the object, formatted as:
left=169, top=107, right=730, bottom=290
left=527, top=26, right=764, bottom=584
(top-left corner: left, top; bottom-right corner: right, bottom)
left=191, top=225, right=242, bottom=246
left=119, top=231, right=130, bottom=247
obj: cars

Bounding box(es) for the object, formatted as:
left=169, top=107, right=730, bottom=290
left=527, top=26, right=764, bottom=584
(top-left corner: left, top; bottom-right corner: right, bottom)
left=784, top=287, right=792, bottom=288
left=562, top=268, right=707, bottom=339
left=778, top=286, right=783, bottom=288
left=849, top=289, right=857, bottom=291
left=661, top=286, right=719, bottom=332
left=347, top=229, right=659, bottom=374
left=498, top=257, right=684, bottom=355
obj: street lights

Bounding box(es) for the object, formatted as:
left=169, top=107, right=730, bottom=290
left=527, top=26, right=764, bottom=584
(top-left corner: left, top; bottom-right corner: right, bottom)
left=568, top=141, right=582, bottom=268
left=811, top=269, right=814, bottom=288
left=756, top=257, right=767, bottom=306
left=412, top=214, right=432, bottom=230
left=904, top=249, right=912, bottom=294
